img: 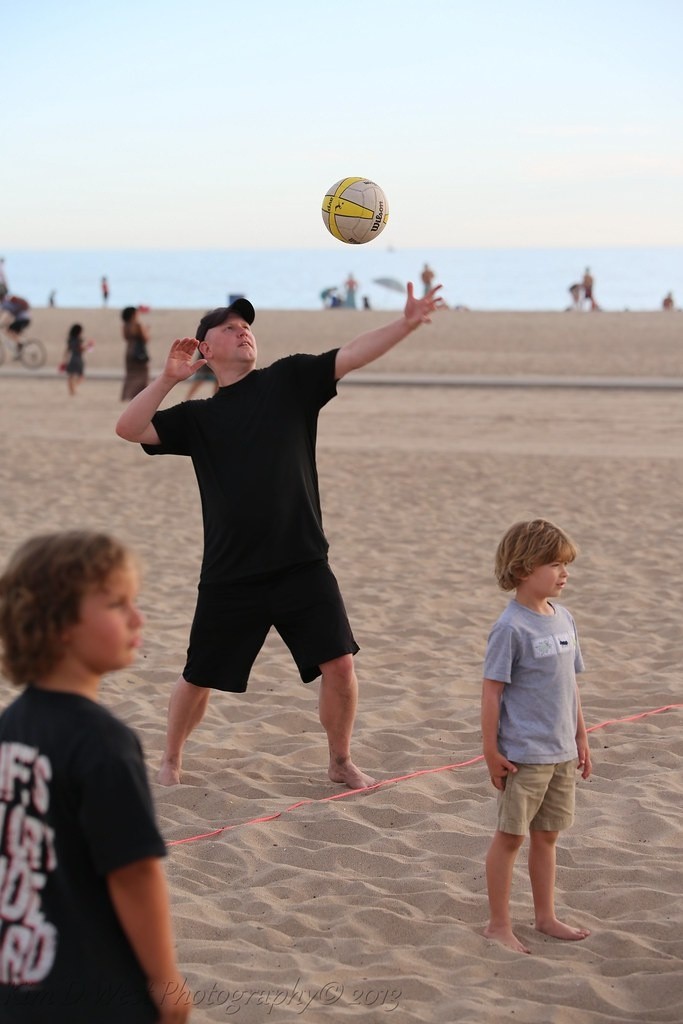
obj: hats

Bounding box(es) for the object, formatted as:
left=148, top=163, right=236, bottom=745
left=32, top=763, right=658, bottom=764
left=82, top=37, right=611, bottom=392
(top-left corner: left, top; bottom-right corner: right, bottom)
left=195, top=298, right=255, bottom=371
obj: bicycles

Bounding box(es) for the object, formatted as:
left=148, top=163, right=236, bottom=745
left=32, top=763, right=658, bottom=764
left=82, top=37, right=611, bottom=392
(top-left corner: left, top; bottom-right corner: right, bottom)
left=1, top=322, right=47, bottom=371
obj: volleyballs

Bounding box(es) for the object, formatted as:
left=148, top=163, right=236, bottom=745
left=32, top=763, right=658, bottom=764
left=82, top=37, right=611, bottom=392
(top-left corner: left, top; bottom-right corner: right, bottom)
left=322, top=176, right=388, bottom=244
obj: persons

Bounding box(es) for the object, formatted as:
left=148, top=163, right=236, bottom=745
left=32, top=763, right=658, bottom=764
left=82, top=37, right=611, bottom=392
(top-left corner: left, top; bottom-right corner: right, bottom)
left=422, top=264, right=435, bottom=296
left=662, top=293, right=674, bottom=311
left=1, top=526, right=200, bottom=1024
left=564, top=284, right=583, bottom=311
left=345, top=272, right=359, bottom=308
left=580, top=267, right=599, bottom=312
left=121, top=307, right=152, bottom=402
left=364, top=297, right=369, bottom=308
left=482, top=521, right=592, bottom=957
left=320, top=286, right=338, bottom=307
left=0, top=257, right=33, bottom=360
left=49, top=291, right=56, bottom=306
left=101, top=276, right=109, bottom=307
left=181, top=352, right=219, bottom=401
left=59, top=324, right=94, bottom=397
left=115, top=284, right=443, bottom=790
left=329, top=292, right=344, bottom=308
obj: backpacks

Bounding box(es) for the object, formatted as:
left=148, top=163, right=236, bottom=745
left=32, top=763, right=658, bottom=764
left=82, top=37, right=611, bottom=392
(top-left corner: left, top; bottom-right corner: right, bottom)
left=11, top=297, right=28, bottom=310
